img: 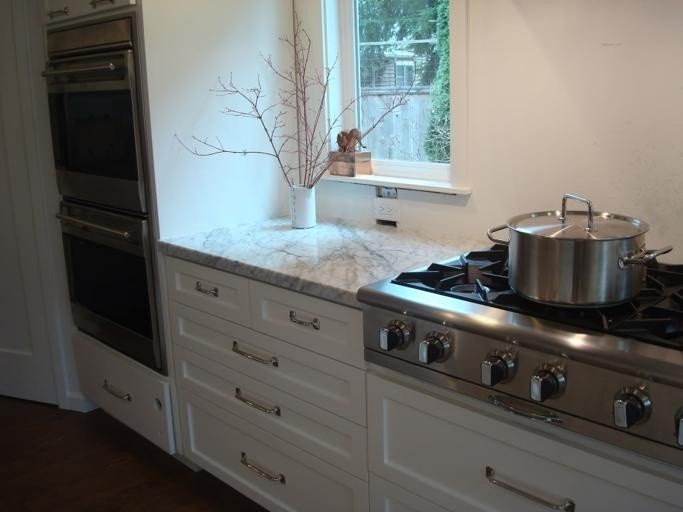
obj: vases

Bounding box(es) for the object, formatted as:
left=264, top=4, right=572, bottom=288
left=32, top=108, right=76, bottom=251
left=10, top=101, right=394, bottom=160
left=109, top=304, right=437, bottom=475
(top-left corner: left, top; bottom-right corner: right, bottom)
left=288, top=185, right=316, bottom=228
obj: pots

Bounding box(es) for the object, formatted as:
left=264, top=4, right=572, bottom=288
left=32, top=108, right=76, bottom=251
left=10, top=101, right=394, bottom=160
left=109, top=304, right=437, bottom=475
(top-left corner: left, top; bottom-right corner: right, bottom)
left=486, top=192, right=674, bottom=308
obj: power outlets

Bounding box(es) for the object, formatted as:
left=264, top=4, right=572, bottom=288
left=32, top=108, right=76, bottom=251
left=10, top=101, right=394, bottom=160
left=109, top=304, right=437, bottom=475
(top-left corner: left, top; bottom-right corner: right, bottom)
left=373, top=199, right=401, bottom=224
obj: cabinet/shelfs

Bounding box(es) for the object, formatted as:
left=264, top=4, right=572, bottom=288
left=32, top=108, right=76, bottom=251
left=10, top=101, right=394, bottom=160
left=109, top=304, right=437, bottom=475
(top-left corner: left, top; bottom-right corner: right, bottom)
left=164, top=254, right=371, bottom=512
left=74, top=330, right=184, bottom=464
left=365, top=365, right=683, bottom=511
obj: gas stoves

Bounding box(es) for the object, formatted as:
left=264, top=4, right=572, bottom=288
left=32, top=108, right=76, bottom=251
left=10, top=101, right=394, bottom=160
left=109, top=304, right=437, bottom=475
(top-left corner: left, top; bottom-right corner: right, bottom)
left=358, top=237, right=683, bottom=447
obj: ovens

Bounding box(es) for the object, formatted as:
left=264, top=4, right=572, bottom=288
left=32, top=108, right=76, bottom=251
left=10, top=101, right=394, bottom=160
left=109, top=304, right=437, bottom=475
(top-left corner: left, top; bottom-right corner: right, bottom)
left=53, top=199, right=166, bottom=376
left=41, top=53, right=152, bottom=219
